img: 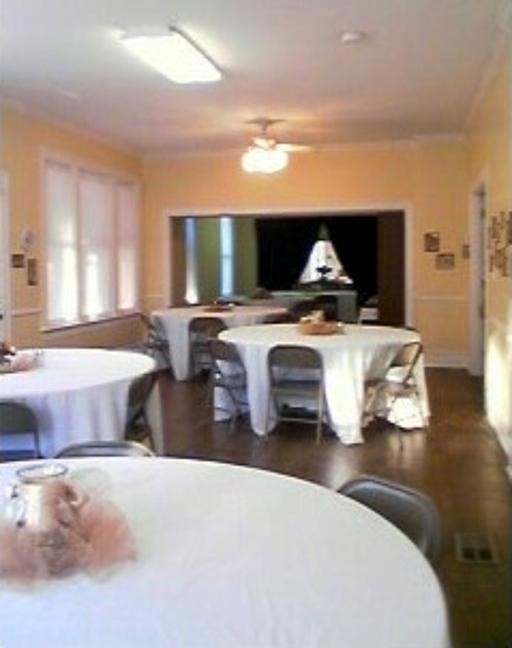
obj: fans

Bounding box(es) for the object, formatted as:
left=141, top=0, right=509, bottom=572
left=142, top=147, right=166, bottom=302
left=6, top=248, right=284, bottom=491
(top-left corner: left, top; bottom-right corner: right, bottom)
left=248, top=119, right=315, bottom=151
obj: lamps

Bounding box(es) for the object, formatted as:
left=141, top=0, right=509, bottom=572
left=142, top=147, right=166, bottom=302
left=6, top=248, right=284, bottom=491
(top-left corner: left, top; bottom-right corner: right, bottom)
left=119, top=25, right=222, bottom=87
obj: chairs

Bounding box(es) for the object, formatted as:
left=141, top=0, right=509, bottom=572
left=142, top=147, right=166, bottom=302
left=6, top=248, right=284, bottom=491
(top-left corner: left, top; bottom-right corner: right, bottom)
left=0, top=400, right=45, bottom=463
left=335, top=472, right=446, bottom=572
left=121, top=348, right=170, bottom=452
left=133, top=291, right=340, bottom=382
left=199, top=322, right=432, bottom=451
left=52, top=439, right=156, bottom=458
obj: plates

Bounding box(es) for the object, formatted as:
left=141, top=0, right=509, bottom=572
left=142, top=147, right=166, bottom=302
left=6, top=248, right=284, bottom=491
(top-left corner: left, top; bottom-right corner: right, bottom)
left=298, top=322, right=342, bottom=333
left=202, top=303, right=235, bottom=312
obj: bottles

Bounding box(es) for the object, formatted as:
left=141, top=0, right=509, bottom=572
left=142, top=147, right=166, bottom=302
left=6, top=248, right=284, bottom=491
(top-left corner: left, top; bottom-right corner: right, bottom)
left=3, top=462, right=92, bottom=575
